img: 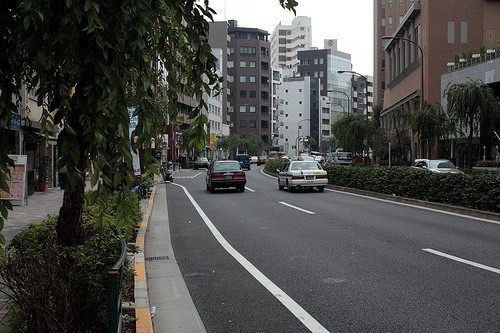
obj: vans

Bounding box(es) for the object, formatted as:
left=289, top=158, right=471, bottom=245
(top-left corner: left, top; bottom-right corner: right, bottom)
left=235, top=154, right=250, bottom=170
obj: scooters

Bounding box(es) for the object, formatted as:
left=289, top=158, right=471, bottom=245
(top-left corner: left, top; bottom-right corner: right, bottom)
left=162, top=167, right=175, bottom=182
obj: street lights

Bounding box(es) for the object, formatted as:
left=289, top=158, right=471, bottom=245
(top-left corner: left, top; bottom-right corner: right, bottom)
left=298, top=118, right=312, bottom=156
left=326, top=102, right=345, bottom=116
left=382, top=36, right=426, bottom=160
left=337, top=71, right=368, bottom=167
left=325, top=90, right=350, bottom=117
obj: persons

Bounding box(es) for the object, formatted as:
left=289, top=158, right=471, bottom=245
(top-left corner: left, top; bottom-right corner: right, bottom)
left=57, top=155, right=68, bottom=189
left=352, top=155, right=363, bottom=163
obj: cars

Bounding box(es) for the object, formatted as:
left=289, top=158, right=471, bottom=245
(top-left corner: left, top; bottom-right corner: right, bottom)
left=250, top=152, right=288, bottom=166
left=411, top=158, right=464, bottom=175
left=206, top=159, right=247, bottom=192
left=277, top=160, right=329, bottom=192
left=195, top=156, right=211, bottom=169
